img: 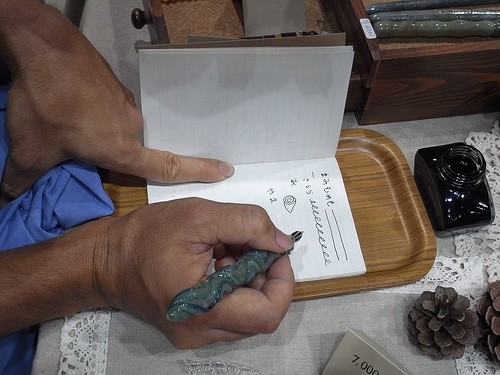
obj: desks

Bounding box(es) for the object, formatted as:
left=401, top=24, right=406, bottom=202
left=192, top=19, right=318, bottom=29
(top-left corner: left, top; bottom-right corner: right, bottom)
left=32, top=0, right=500, bottom=375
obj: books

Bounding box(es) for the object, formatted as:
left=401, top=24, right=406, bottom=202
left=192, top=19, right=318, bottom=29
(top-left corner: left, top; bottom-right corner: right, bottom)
left=136, top=32, right=368, bottom=284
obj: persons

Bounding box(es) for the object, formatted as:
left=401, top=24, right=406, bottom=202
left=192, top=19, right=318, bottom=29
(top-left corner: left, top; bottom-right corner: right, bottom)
left=0, top=0, right=297, bottom=375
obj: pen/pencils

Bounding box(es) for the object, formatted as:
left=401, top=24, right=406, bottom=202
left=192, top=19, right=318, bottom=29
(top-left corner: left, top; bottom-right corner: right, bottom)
left=165, top=230, right=305, bottom=323
left=366, top=1, right=500, bottom=21
left=374, top=22, right=500, bottom=38
